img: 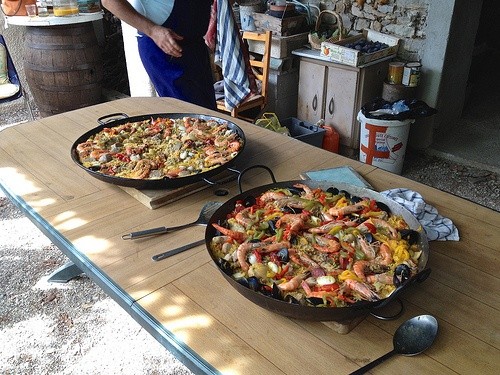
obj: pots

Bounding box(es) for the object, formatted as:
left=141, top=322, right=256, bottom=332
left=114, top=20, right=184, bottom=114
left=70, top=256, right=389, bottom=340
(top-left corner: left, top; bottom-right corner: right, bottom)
left=203, top=163, right=432, bottom=322
left=68, top=111, right=248, bottom=189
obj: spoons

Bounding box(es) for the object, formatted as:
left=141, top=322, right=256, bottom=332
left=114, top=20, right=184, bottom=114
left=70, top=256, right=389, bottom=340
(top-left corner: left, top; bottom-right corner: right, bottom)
left=346, top=315, right=439, bottom=375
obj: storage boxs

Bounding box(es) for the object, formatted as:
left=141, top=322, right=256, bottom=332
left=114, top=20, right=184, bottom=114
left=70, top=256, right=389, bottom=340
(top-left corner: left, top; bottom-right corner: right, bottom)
left=256, top=116, right=326, bottom=148
left=319, top=28, right=402, bottom=68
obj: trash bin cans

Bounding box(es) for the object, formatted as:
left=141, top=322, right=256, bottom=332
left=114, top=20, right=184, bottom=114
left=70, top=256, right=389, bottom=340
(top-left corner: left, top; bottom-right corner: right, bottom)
left=407, top=98, right=438, bottom=150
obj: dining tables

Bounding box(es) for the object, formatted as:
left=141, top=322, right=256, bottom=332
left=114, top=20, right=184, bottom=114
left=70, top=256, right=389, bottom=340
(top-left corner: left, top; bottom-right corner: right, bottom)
left=0, top=95, right=500, bottom=375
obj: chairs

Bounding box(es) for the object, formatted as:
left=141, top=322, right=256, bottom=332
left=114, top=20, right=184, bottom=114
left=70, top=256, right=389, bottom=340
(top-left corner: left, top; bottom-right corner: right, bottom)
left=216, top=29, right=272, bottom=124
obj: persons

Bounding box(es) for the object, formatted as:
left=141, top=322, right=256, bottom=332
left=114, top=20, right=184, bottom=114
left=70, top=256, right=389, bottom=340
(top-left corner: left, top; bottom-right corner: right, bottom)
left=102, top=0, right=217, bottom=112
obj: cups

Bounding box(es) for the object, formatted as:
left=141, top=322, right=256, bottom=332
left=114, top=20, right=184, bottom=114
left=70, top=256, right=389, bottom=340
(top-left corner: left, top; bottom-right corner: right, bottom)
left=24, top=0, right=82, bottom=16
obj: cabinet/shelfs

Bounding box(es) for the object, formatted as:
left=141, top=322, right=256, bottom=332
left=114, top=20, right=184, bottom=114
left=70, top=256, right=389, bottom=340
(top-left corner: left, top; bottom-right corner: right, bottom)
left=292, top=48, right=398, bottom=156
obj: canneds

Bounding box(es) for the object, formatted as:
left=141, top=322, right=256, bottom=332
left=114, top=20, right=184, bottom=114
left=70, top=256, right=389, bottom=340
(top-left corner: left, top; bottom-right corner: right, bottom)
left=402, top=62, right=421, bottom=87
left=388, top=62, right=405, bottom=84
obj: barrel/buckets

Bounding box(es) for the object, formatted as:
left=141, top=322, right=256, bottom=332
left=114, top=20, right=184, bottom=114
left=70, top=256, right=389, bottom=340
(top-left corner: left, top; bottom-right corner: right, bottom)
left=23, top=21, right=103, bottom=119
